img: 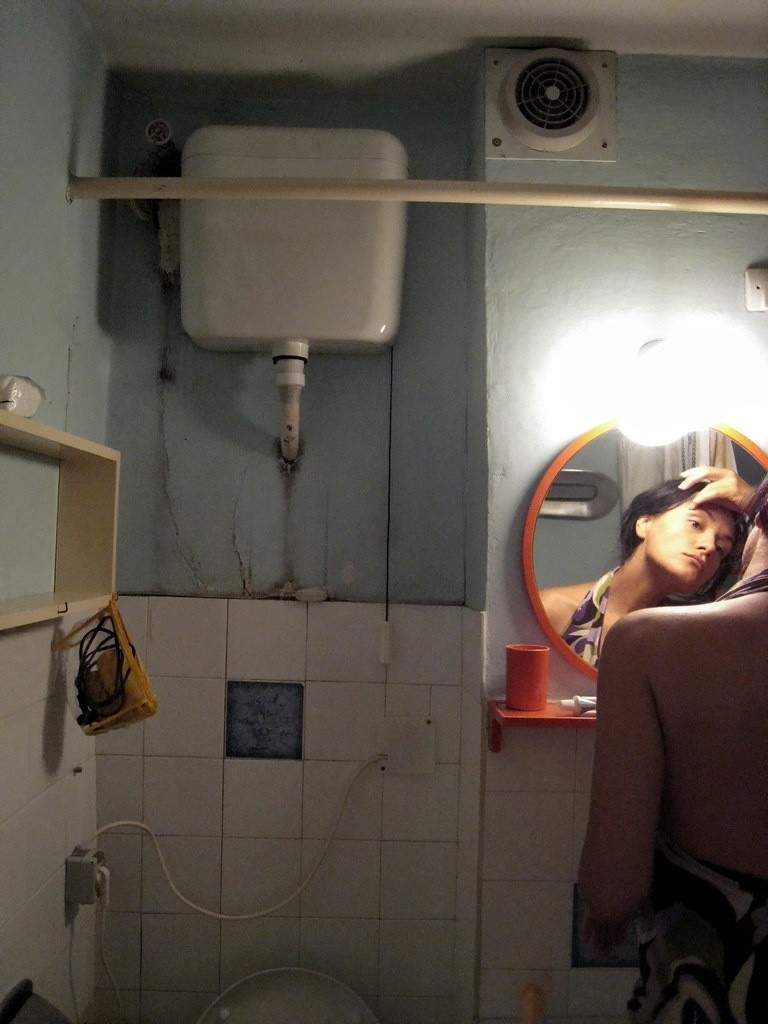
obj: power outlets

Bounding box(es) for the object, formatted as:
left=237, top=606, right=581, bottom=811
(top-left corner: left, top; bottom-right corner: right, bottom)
left=65, top=850, right=106, bottom=905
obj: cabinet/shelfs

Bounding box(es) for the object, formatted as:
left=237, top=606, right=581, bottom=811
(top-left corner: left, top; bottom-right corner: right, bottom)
left=0, top=409, right=122, bottom=632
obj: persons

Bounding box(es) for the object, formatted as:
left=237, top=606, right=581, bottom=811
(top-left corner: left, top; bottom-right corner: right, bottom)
left=536, top=465, right=758, bottom=671
left=571, top=473, right=768, bottom=1024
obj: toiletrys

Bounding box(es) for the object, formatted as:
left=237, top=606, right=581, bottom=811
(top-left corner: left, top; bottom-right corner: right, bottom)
left=558, top=696, right=596, bottom=715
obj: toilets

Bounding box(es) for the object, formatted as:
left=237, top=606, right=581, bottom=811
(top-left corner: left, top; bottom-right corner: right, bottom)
left=196, top=966, right=380, bottom=1024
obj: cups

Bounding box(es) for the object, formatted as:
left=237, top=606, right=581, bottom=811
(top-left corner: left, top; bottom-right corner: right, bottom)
left=506, top=643, right=550, bottom=711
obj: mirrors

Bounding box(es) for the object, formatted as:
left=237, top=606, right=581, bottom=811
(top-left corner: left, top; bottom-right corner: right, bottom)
left=521, top=424, right=768, bottom=679
